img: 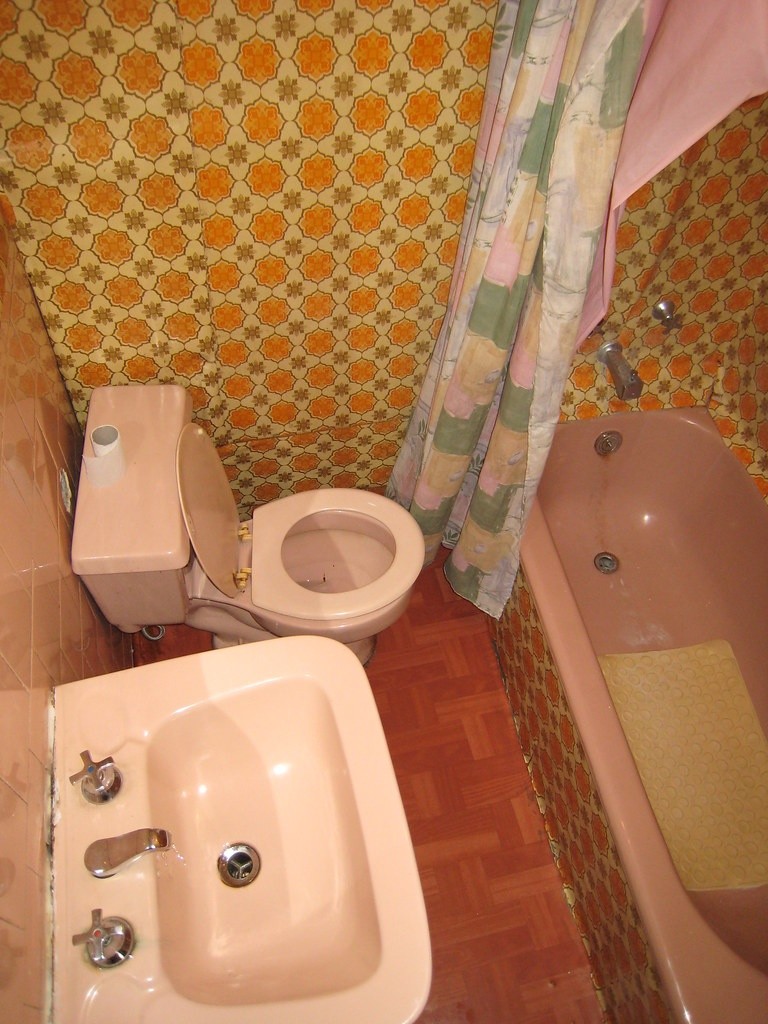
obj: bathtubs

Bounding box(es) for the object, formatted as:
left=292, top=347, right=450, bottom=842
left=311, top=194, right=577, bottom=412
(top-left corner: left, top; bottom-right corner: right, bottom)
left=482, top=408, right=764, bottom=1024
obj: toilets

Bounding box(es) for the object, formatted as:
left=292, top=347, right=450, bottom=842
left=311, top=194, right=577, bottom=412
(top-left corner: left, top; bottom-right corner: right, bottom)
left=68, top=384, right=426, bottom=671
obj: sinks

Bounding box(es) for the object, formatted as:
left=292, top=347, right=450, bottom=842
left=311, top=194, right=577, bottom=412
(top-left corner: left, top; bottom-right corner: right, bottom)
left=49, top=634, right=435, bottom=1023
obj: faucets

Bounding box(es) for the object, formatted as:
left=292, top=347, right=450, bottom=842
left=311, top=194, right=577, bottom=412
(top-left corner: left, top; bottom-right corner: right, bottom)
left=596, top=341, right=644, bottom=402
left=83, top=828, right=173, bottom=879
